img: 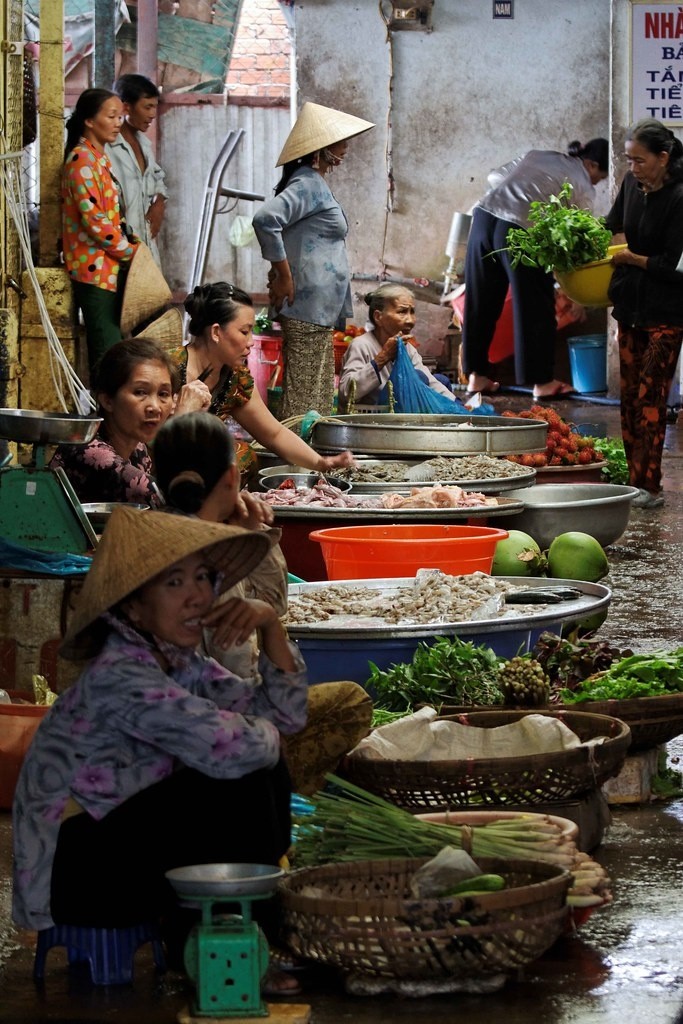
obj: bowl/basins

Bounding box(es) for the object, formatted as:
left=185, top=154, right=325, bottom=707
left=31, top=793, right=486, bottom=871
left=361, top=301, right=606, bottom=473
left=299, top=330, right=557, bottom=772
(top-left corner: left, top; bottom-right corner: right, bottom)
left=413, top=811, right=580, bottom=840
left=258, top=473, right=353, bottom=494
left=534, top=458, right=609, bottom=483
left=551, top=243, right=628, bottom=307
left=290, top=622, right=613, bottom=701
left=492, top=484, right=640, bottom=551
left=80, top=502, right=152, bottom=532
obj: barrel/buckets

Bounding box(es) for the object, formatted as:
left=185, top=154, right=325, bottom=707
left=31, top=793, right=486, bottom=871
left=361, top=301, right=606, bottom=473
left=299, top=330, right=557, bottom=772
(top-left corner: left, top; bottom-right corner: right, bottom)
left=0, top=689, right=52, bottom=809
left=566, top=333, right=608, bottom=393
left=310, top=524, right=510, bottom=581
left=246, top=334, right=284, bottom=408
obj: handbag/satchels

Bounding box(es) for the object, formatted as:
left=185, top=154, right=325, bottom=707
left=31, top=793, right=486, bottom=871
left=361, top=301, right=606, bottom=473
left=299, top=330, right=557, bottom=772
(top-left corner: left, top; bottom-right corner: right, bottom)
left=377, top=337, right=498, bottom=416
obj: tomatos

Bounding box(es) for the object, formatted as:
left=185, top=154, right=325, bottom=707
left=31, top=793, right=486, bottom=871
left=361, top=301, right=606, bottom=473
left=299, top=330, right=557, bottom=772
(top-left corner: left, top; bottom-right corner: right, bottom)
left=331, top=324, right=367, bottom=344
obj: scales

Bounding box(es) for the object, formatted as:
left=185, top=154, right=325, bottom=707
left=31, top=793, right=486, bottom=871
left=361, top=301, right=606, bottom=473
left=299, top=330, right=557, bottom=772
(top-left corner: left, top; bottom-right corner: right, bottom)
left=0, top=408, right=105, bottom=560
left=165, top=863, right=288, bottom=1017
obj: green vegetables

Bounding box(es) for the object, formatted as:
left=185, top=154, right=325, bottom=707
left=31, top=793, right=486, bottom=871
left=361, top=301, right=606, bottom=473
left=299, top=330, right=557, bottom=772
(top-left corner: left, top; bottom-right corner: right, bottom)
left=583, top=436, right=630, bottom=487
left=481, top=180, right=613, bottom=277
left=362, top=633, right=683, bottom=707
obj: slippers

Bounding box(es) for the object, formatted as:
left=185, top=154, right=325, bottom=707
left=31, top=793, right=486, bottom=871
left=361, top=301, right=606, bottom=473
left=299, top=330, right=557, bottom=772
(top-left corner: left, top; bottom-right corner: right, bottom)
left=466, top=379, right=500, bottom=396
left=532, top=382, right=581, bottom=400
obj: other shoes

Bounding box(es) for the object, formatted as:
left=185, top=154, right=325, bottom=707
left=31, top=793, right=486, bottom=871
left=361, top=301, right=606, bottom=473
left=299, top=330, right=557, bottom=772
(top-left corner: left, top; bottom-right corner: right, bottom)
left=635, top=488, right=667, bottom=507
left=261, top=962, right=300, bottom=1003
left=266, top=947, right=304, bottom=972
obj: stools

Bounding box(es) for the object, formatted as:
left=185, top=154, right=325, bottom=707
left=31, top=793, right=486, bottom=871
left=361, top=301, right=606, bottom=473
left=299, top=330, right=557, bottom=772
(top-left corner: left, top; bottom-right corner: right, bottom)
left=29, top=917, right=169, bottom=988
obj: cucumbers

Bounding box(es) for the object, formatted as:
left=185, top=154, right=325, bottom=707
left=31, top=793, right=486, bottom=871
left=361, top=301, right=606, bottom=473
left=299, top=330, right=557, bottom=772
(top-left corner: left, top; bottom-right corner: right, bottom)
left=438, top=874, right=505, bottom=897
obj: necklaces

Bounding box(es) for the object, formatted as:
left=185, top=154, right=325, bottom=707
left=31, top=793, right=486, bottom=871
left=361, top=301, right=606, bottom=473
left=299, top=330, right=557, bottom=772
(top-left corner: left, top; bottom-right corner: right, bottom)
left=194, top=346, right=218, bottom=379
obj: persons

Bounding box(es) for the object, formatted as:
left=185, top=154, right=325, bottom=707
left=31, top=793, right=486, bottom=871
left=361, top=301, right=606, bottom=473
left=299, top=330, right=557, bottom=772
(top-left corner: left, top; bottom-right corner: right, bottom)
left=13, top=508, right=311, bottom=995
left=47, top=337, right=276, bottom=527
left=251, top=102, right=375, bottom=425
left=341, top=281, right=464, bottom=409
left=599, top=117, right=683, bottom=508
left=160, top=282, right=355, bottom=473
left=462, top=137, right=609, bottom=401
left=105, top=72, right=169, bottom=274
left=148, top=408, right=288, bottom=682
left=59, top=86, right=142, bottom=384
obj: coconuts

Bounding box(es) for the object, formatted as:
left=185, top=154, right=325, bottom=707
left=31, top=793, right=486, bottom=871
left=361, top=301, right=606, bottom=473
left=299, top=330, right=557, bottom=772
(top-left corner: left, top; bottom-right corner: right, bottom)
left=491, top=529, right=611, bottom=584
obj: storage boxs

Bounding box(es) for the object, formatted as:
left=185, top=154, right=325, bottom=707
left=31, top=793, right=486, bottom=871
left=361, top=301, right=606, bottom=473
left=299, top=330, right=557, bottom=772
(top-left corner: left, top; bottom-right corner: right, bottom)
left=604, top=747, right=669, bottom=805
left=526, top=800, right=610, bottom=858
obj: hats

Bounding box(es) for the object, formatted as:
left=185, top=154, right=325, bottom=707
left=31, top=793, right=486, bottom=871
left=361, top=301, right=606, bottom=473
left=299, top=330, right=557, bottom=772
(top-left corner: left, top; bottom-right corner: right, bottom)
left=56, top=505, right=271, bottom=662
left=134, top=308, right=183, bottom=348
left=121, top=242, right=174, bottom=337
left=275, top=101, right=375, bottom=169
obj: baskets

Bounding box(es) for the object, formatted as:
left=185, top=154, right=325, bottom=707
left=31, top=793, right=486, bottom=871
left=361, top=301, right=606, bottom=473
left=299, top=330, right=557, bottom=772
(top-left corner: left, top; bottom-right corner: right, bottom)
left=338, top=708, right=632, bottom=808
left=279, top=854, right=576, bottom=979
left=410, top=691, right=683, bottom=753
